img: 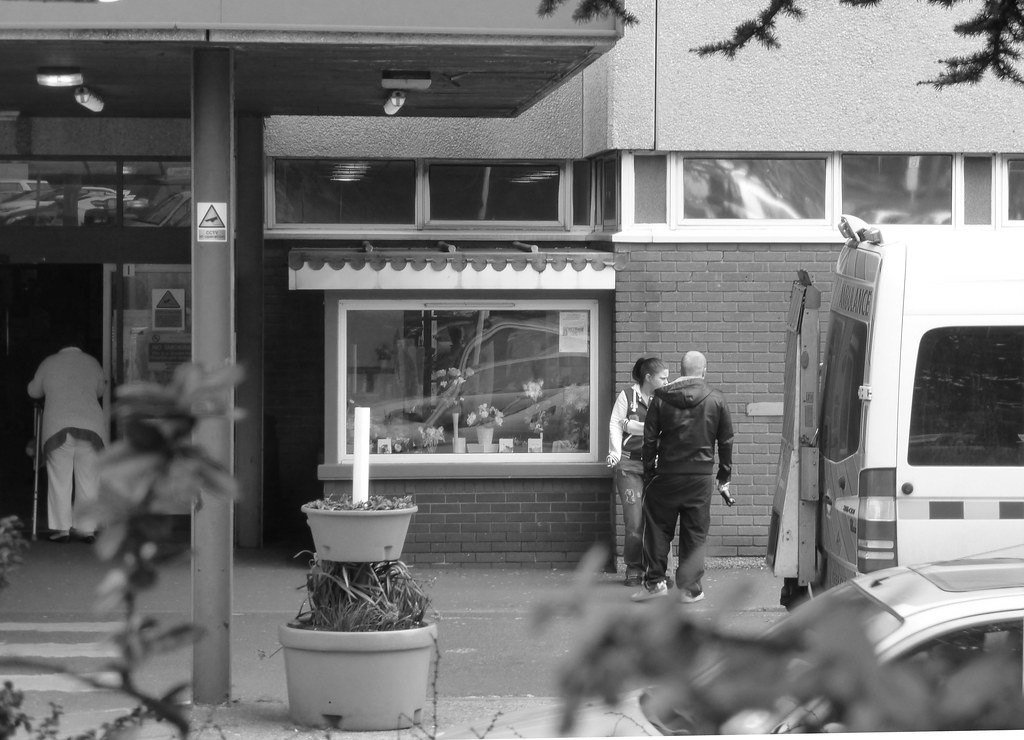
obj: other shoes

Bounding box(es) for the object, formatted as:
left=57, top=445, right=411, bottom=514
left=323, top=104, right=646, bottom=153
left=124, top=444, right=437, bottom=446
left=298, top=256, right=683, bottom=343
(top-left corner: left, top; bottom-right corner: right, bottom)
left=626, top=567, right=643, bottom=586
left=665, top=576, right=674, bottom=589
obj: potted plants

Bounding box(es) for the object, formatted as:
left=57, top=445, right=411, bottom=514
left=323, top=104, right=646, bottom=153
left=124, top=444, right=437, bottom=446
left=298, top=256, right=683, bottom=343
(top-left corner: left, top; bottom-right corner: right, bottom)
left=301, top=496, right=419, bottom=564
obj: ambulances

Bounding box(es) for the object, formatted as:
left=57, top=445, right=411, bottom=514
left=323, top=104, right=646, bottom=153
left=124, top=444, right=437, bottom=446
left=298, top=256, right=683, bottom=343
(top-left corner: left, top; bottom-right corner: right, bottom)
left=766, top=214, right=1024, bottom=612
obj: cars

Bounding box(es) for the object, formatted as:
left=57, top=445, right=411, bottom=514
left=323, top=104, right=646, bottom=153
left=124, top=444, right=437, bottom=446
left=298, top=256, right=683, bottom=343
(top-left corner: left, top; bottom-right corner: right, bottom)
left=429, top=527, right=1023, bottom=739
left=361, top=305, right=592, bottom=444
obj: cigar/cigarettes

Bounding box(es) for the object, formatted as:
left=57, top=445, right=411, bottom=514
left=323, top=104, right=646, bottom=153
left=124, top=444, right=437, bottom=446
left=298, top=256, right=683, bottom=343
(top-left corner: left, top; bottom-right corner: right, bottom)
left=607, top=465, right=613, bottom=468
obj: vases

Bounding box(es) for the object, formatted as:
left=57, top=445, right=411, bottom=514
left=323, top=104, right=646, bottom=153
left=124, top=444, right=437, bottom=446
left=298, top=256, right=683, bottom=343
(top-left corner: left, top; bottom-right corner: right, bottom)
left=451, top=413, right=459, bottom=453
left=476, top=428, right=494, bottom=445
left=279, top=616, right=437, bottom=730
left=467, top=444, right=499, bottom=453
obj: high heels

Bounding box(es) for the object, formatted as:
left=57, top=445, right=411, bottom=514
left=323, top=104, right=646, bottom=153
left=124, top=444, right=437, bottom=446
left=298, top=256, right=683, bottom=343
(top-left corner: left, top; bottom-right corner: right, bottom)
left=50, top=532, right=70, bottom=543
left=84, top=533, right=95, bottom=544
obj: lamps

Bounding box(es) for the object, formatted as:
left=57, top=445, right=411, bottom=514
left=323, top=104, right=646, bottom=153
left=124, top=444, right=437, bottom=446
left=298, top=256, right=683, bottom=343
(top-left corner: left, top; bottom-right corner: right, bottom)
left=74, top=87, right=105, bottom=112
left=36, top=67, right=83, bottom=87
left=382, top=70, right=432, bottom=91
left=383, top=92, right=406, bottom=116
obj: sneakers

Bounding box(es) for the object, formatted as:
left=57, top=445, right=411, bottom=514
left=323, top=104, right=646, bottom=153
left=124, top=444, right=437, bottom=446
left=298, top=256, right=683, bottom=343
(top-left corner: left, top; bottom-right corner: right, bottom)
left=630, top=580, right=667, bottom=601
left=681, top=591, right=705, bottom=602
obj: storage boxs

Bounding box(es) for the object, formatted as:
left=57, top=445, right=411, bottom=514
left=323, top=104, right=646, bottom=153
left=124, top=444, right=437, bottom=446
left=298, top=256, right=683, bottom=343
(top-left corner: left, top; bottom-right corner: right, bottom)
left=498, top=437, right=515, bottom=453
left=528, top=437, right=543, bottom=453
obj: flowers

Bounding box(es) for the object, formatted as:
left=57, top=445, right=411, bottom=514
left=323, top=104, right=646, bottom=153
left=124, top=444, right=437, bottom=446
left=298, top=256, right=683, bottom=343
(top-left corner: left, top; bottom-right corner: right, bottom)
left=435, top=367, right=474, bottom=412
left=523, top=377, right=548, bottom=433
left=466, top=403, right=504, bottom=429
left=418, top=425, right=446, bottom=454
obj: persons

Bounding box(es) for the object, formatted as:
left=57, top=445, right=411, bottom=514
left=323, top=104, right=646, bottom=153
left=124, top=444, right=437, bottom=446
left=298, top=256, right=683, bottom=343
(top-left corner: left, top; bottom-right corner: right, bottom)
left=632, top=351, right=733, bottom=604
left=28, top=335, right=105, bottom=543
left=606, top=358, right=675, bottom=589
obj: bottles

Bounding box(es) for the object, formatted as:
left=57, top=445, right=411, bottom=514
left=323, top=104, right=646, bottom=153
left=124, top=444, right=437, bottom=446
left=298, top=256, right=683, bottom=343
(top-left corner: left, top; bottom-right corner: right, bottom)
left=718, top=484, right=735, bottom=507
